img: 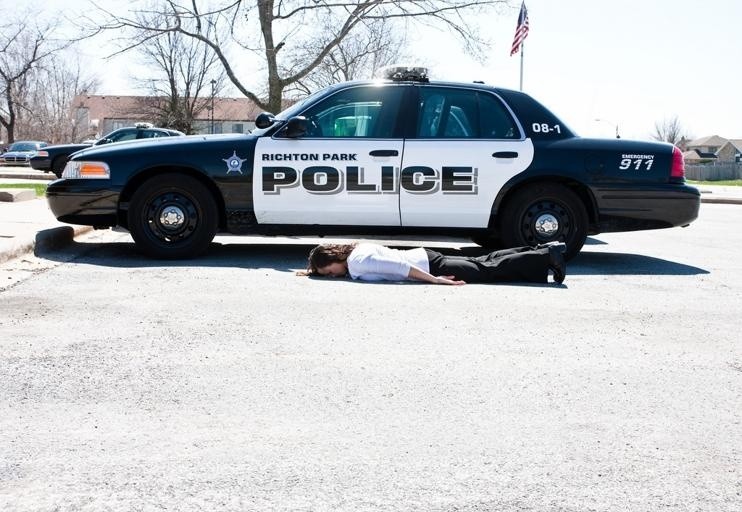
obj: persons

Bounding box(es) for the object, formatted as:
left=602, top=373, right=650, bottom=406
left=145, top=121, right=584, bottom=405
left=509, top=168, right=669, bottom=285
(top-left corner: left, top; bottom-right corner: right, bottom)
left=295, top=240, right=568, bottom=286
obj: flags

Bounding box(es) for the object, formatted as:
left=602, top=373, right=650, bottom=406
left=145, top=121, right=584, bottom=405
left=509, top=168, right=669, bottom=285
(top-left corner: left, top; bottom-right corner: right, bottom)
left=509, top=0, right=530, bottom=56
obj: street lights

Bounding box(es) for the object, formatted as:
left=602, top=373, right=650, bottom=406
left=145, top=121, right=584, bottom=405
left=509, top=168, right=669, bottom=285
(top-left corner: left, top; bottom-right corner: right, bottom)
left=596, top=118, right=619, bottom=137
left=205, top=105, right=212, bottom=134
left=210, top=78, right=217, bottom=134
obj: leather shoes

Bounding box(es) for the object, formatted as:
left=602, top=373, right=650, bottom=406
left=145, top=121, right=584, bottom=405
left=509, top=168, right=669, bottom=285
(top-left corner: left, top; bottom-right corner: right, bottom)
left=547, top=241, right=567, bottom=284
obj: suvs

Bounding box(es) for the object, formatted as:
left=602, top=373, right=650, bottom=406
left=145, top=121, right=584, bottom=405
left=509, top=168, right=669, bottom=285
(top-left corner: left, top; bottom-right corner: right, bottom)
left=0, top=141, right=48, bottom=167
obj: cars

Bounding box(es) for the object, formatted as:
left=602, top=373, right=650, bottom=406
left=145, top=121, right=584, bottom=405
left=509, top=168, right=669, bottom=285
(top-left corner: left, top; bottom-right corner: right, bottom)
left=30, top=122, right=187, bottom=179
left=70, top=139, right=98, bottom=145
left=46, top=66, right=701, bottom=266
